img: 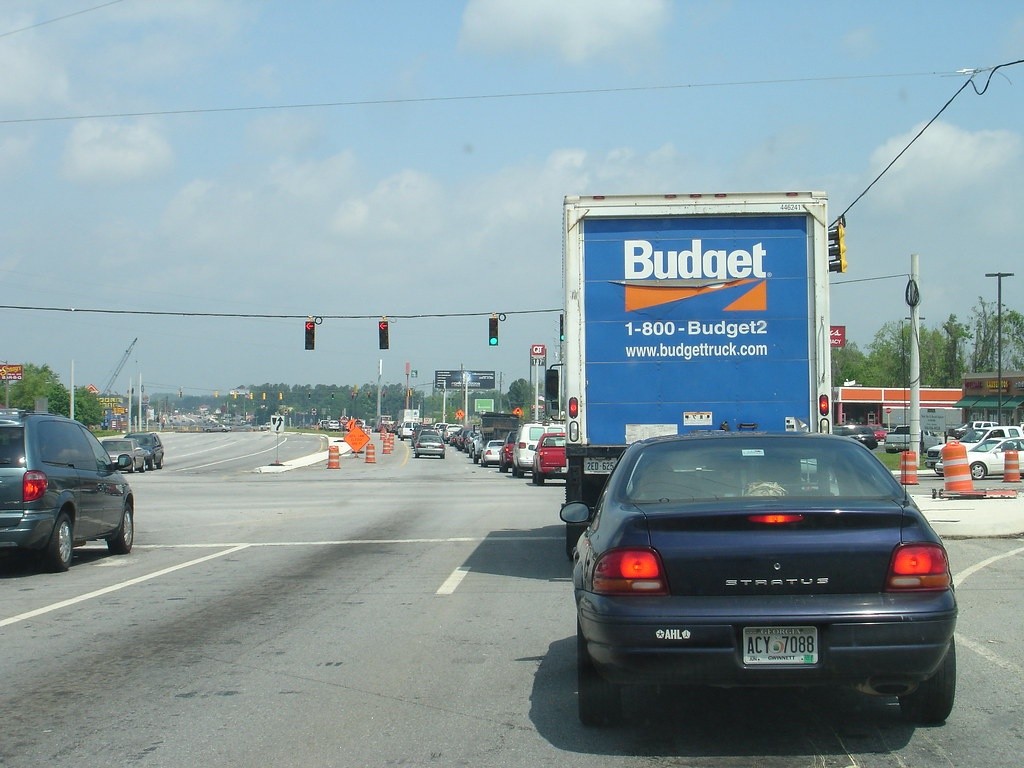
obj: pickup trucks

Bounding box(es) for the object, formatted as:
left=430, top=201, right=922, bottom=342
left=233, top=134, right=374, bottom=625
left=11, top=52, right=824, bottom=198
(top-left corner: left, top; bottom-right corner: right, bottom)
left=884, top=425, right=942, bottom=453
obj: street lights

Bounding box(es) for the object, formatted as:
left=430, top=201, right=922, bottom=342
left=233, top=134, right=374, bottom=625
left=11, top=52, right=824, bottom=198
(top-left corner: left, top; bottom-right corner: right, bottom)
left=986, top=272, right=1014, bottom=424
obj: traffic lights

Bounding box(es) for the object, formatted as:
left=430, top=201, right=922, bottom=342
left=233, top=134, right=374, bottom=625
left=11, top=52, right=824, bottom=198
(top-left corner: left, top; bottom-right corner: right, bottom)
left=379, top=321, right=389, bottom=349
left=560, top=313, right=563, bottom=341
left=489, top=318, right=498, bottom=346
left=304, top=321, right=315, bottom=351
left=828, top=223, right=848, bottom=273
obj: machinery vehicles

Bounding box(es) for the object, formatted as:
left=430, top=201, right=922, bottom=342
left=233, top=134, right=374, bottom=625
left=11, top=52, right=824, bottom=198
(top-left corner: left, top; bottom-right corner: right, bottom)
left=92, top=337, right=138, bottom=395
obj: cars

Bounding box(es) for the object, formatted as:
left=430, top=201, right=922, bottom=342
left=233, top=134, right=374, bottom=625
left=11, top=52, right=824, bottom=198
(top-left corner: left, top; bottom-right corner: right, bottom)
left=434, top=412, right=567, bottom=486
left=205, top=424, right=232, bottom=432
left=924, top=420, right=1024, bottom=480
left=559, top=430, right=958, bottom=727
left=397, top=420, right=446, bottom=459
left=102, top=432, right=165, bottom=473
left=0, top=408, right=134, bottom=570
left=318, top=419, right=340, bottom=431
left=833, top=418, right=885, bottom=449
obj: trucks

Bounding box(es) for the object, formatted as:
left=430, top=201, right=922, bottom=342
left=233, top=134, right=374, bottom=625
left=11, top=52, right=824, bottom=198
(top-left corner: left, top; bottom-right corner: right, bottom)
left=544, top=190, right=833, bottom=561
left=398, top=409, right=420, bottom=422
